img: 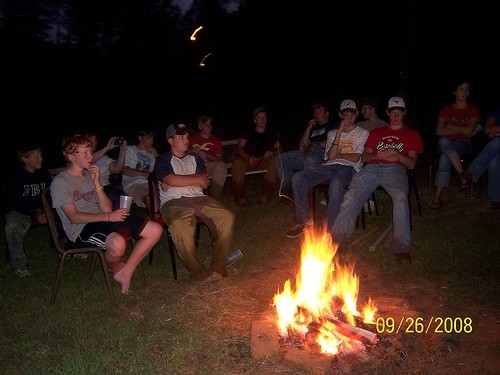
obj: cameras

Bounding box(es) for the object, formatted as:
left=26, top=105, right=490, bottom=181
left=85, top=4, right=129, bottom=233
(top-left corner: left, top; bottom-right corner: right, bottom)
left=114, top=138, right=123, bottom=145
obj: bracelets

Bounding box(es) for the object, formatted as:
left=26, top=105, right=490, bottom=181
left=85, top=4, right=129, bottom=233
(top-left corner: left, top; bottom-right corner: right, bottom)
left=332, top=142, right=339, bottom=145
left=107, top=212, right=110, bottom=223
left=95, top=185, right=104, bottom=192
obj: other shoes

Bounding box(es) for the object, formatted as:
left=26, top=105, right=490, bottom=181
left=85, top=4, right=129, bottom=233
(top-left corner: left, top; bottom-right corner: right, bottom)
left=394, top=252, right=411, bottom=265
left=365, top=200, right=375, bottom=212
left=321, top=199, right=327, bottom=205
left=59, top=253, right=88, bottom=260
left=273, top=193, right=293, bottom=205
left=238, top=198, right=251, bottom=207
left=258, top=192, right=271, bottom=204
left=286, top=223, right=305, bottom=237
left=15, top=268, right=30, bottom=277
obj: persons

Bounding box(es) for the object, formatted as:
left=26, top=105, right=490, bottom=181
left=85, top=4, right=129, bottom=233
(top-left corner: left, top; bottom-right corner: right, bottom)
left=427, top=79, right=481, bottom=213
left=329, top=96, right=426, bottom=268
left=6, top=97, right=391, bottom=295
left=462, top=105, right=500, bottom=212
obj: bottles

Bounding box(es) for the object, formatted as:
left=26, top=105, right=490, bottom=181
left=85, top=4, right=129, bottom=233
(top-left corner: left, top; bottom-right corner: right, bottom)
left=225, top=248, right=245, bottom=264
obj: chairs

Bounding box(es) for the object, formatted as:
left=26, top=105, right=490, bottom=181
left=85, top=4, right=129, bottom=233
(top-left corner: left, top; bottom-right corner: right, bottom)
left=311, top=132, right=482, bottom=232
left=39, top=173, right=207, bottom=310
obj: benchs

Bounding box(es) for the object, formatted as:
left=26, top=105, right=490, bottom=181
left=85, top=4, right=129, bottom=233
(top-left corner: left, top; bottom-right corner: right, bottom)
left=207, top=139, right=269, bottom=180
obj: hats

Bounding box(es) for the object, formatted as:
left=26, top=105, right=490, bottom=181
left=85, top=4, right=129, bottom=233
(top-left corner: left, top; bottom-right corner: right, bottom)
left=387, top=97, right=406, bottom=113
left=340, top=99, right=357, bottom=114
left=166, top=122, right=192, bottom=139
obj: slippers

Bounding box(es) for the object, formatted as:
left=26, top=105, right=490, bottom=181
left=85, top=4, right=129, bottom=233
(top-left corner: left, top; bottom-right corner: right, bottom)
left=490, top=201, right=500, bottom=209
left=459, top=172, right=472, bottom=183
left=430, top=201, right=440, bottom=209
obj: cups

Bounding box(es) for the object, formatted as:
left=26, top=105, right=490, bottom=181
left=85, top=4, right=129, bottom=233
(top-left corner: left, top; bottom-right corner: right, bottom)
left=120, top=195, right=133, bottom=212
